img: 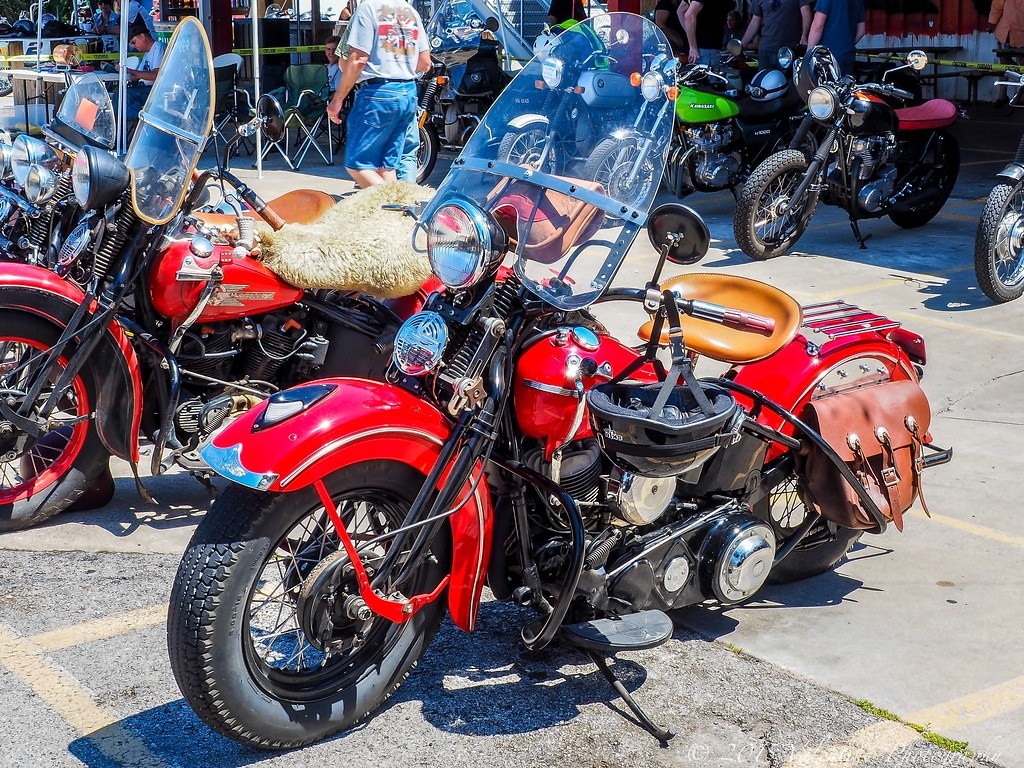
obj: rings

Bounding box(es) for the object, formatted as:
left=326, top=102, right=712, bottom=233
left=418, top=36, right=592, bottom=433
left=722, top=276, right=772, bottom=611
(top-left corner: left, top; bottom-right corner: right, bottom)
left=331, top=117, right=334, bottom=119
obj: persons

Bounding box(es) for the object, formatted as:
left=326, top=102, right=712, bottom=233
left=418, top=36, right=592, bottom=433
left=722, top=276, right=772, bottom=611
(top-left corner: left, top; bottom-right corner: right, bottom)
left=325, top=0, right=432, bottom=186
left=548, top=0, right=868, bottom=78
left=91, top=0, right=167, bottom=132
left=986, top=0, right=1024, bottom=106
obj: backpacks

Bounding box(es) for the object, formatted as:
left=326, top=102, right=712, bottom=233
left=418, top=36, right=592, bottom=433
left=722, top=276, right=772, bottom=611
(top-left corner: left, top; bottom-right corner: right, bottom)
left=43, top=20, right=84, bottom=37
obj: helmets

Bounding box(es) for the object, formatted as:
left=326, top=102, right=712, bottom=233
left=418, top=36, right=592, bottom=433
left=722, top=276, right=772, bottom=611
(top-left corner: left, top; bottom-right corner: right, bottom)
left=792, top=45, right=840, bottom=103
left=36, top=13, right=59, bottom=30
left=749, top=68, right=789, bottom=102
left=19, top=430, right=115, bottom=512
left=587, top=382, right=745, bottom=480
left=0, top=20, right=12, bottom=37
left=12, top=19, right=36, bottom=35
left=533, top=33, right=562, bottom=62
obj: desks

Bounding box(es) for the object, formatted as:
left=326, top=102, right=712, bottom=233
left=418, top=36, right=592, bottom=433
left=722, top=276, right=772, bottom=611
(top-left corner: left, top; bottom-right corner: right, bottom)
left=0, top=36, right=104, bottom=80
left=0, top=69, right=132, bottom=146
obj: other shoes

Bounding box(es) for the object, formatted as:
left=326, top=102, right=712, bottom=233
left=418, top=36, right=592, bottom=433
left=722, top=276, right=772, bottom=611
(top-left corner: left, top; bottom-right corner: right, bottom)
left=994, top=97, right=1010, bottom=107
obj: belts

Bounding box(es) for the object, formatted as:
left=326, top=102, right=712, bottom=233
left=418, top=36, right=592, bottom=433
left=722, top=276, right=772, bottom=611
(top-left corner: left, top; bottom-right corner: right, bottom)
left=367, top=78, right=413, bottom=84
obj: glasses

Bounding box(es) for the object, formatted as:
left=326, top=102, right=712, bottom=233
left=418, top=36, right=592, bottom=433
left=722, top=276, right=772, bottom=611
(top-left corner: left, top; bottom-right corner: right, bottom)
left=130, top=35, right=140, bottom=45
left=99, top=2, right=109, bottom=5
left=744, top=82, right=765, bottom=98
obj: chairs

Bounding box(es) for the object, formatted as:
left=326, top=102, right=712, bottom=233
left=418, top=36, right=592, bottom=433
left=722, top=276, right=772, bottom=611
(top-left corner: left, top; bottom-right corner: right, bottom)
left=101, top=53, right=356, bottom=171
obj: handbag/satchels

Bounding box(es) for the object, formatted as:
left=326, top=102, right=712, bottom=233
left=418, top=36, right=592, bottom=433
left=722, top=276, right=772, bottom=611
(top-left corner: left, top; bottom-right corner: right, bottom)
left=552, top=19, right=609, bottom=69
left=803, top=379, right=931, bottom=530
left=483, top=165, right=607, bottom=262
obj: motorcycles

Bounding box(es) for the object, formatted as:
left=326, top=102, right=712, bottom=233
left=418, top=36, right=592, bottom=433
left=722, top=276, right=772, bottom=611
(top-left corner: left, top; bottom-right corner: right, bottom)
left=733, top=40, right=960, bottom=260
left=0, top=16, right=572, bottom=535
left=165, top=10, right=953, bottom=750
left=582, top=21, right=820, bottom=228
left=400, top=0, right=637, bottom=185
left=973, top=68, right=1024, bottom=301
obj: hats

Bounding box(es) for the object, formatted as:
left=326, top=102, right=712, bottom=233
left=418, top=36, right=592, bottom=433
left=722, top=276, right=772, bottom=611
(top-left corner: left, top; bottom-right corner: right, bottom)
left=128, top=26, right=149, bottom=41
